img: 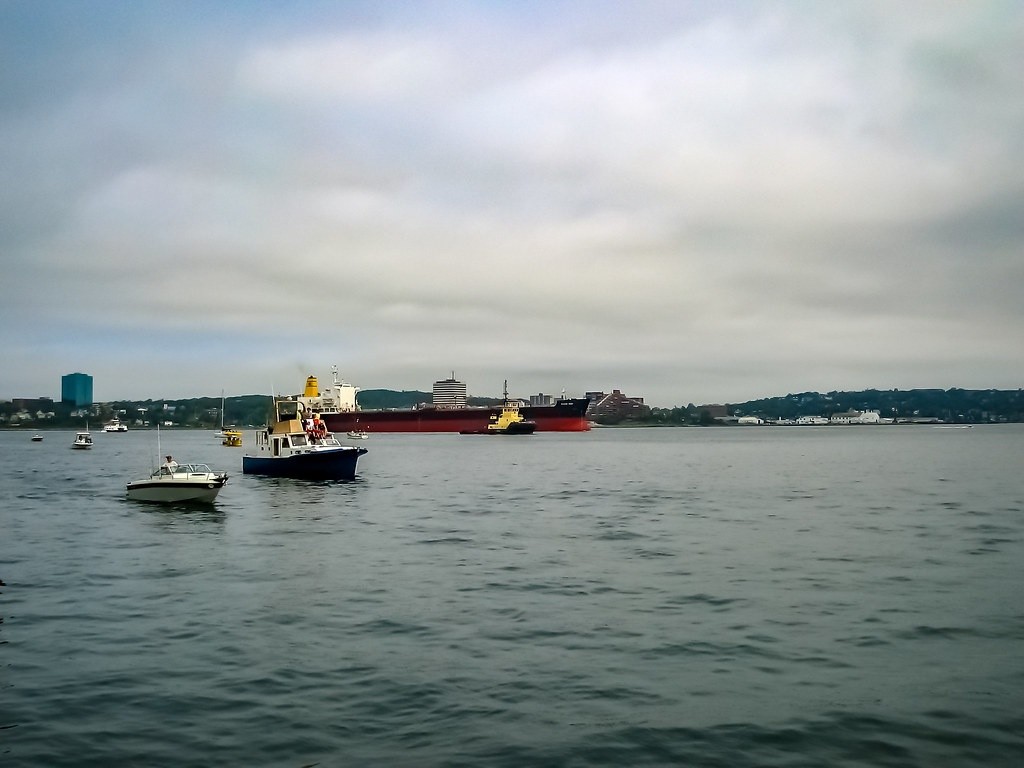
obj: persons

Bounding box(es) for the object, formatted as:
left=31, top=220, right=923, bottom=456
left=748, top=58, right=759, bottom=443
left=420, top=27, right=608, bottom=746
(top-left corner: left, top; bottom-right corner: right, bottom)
left=163, top=456, right=178, bottom=473
left=304, top=407, right=326, bottom=444
left=79, top=435, right=90, bottom=443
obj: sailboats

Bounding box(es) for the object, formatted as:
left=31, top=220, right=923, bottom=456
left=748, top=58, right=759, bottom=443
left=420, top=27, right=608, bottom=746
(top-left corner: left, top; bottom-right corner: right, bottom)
left=214, top=387, right=235, bottom=438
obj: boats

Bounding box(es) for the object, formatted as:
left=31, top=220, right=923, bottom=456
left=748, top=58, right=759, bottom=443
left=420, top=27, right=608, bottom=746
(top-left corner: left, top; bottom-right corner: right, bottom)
left=32, top=434, right=44, bottom=441
left=222, top=436, right=242, bottom=447
left=459, top=380, right=537, bottom=436
left=72, top=433, right=94, bottom=449
left=225, top=430, right=242, bottom=436
left=124, top=462, right=230, bottom=506
left=346, top=431, right=368, bottom=439
left=241, top=401, right=369, bottom=484
left=278, top=374, right=593, bottom=432
left=103, top=417, right=128, bottom=433
